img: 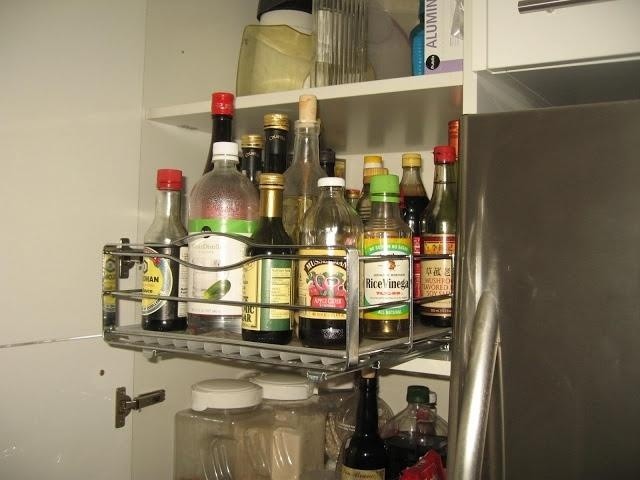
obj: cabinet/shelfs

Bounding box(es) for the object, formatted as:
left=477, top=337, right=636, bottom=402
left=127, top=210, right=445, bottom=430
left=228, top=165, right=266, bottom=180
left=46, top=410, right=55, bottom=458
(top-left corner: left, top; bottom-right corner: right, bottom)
left=135, top=1, right=478, bottom=381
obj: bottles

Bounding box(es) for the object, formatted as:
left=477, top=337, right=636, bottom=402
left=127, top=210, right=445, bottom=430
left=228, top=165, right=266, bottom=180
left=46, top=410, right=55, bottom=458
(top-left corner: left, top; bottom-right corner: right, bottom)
left=419, top=393, right=438, bottom=434
left=239, top=134, right=266, bottom=171
left=282, top=96, right=333, bottom=243
left=174, top=378, right=272, bottom=480
left=363, top=156, right=383, bottom=178
left=205, top=92, right=241, bottom=172
left=417, top=145, right=457, bottom=328
left=261, top=115, right=292, bottom=175
left=317, top=147, right=335, bottom=178
left=244, top=167, right=294, bottom=344
left=362, top=175, right=413, bottom=341
left=307, top=369, right=327, bottom=394
left=188, top=141, right=258, bottom=317
left=296, top=177, right=361, bottom=349
left=347, top=187, right=361, bottom=210
left=381, top=385, right=448, bottom=479
left=250, top=372, right=325, bottom=479
left=448, top=118, right=461, bottom=199
left=326, top=369, right=395, bottom=480
left=411, top=2, right=425, bottom=76
left=400, top=153, right=435, bottom=232
left=344, top=370, right=388, bottom=479
left=252, top=2, right=316, bottom=94
left=142, top=169, right=187, bottom=331
left=356, top=168, right=392, bottom=224
